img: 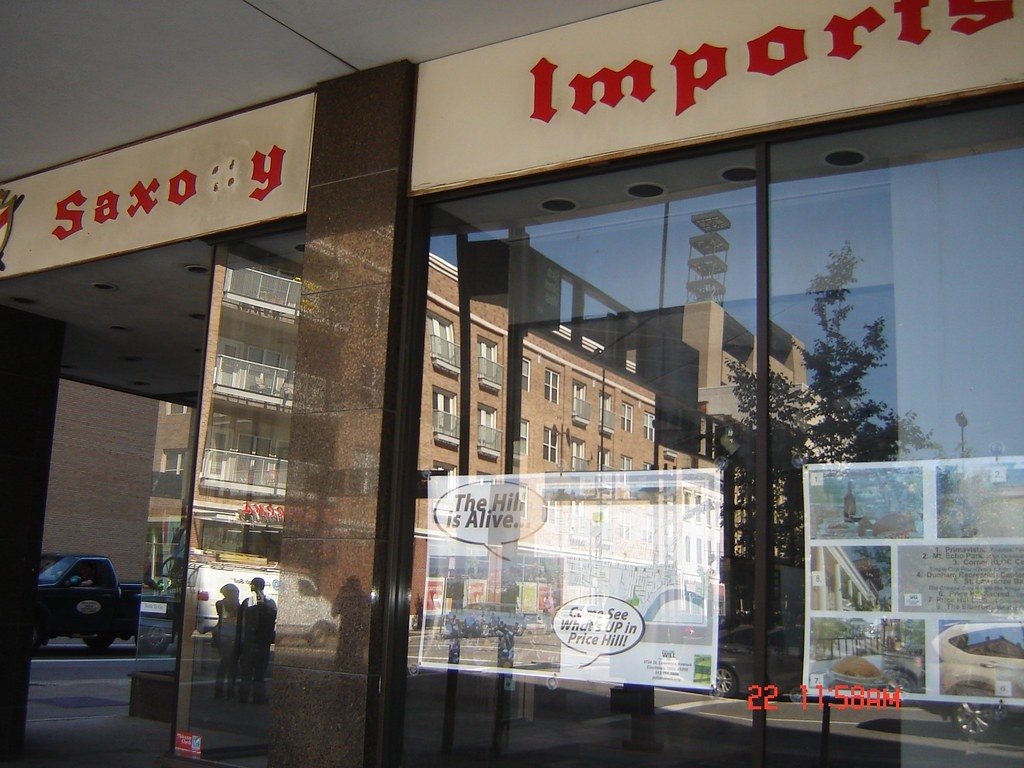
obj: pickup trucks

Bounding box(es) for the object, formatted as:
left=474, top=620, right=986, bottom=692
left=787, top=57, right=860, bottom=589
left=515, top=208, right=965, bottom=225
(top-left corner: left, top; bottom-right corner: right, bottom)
left=34, top=553, right=179, bottom=659
left=861, top=639, right=920, bottom=694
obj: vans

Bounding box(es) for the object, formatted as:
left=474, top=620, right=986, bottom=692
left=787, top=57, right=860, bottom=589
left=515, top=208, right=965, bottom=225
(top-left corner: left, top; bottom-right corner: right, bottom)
left=192, top=557, right=344, bottom=649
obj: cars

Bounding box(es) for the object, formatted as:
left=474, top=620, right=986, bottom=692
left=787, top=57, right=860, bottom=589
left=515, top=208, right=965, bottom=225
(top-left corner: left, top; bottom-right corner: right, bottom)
left=412, top=597, right=451, bottom=627
left=447, top=604, right=529, bottom=636
left=708, top=620, right=803, bottom=699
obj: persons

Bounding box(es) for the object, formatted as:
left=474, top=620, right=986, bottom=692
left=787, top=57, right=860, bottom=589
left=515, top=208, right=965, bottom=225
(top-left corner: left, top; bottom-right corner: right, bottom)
left=236, top=578, right=277, bottom=704
left=212, top=583, right=240, bottom=698
left=442, top=612, right=522, bottom=653
left=330, top=574, right=368, bottom=671
left=65, top=560, right=97, bottom=587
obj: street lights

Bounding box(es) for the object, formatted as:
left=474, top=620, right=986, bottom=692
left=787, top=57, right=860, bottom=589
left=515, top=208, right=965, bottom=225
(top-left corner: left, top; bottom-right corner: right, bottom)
left=955, top=412, right=971, bottom=473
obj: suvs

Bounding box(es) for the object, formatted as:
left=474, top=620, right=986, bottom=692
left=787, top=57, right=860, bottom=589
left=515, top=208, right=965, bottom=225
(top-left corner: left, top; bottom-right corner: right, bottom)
left=923, top=620, right=1024, bottom=740
